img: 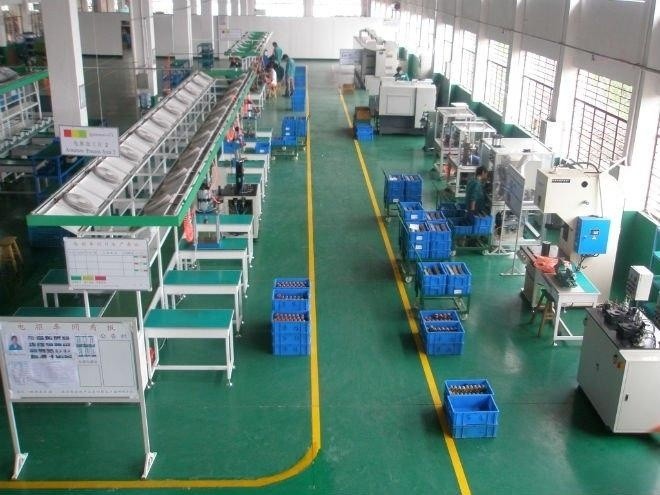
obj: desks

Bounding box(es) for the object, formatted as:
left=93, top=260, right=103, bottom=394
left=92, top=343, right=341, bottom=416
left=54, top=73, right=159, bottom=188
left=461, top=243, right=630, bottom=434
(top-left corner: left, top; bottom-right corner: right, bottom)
left=12, top=268, right=104, bottom=318
left=541, top=260, right=601, bottom=347
left=142, top=131, right=272, bottom=385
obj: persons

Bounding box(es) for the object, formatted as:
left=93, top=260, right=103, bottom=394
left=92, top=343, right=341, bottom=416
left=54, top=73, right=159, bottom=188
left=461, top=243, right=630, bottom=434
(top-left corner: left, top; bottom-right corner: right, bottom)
left=466, top=167, right=488, bottom=214
left=282, top=54, right=295, bottom=97
left=272, top=42, right=282, bottom=63
left=229, top=57, right=241, bottom=68
left=266, top=64, right=277, bottom=87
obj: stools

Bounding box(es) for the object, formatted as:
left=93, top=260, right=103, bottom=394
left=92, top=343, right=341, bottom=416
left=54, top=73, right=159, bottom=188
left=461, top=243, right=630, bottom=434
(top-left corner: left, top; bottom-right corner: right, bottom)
left=531, top=289, right=561, bottom=339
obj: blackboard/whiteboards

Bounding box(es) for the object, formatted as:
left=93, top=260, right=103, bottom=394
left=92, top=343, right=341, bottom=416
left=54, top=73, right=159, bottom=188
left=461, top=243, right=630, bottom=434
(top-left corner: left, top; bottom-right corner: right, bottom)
left=0, top=316, right=145, bottom=403
left=59, top=125, right=120, bottom=157
left=63, top=237, right=152, bottom=292
